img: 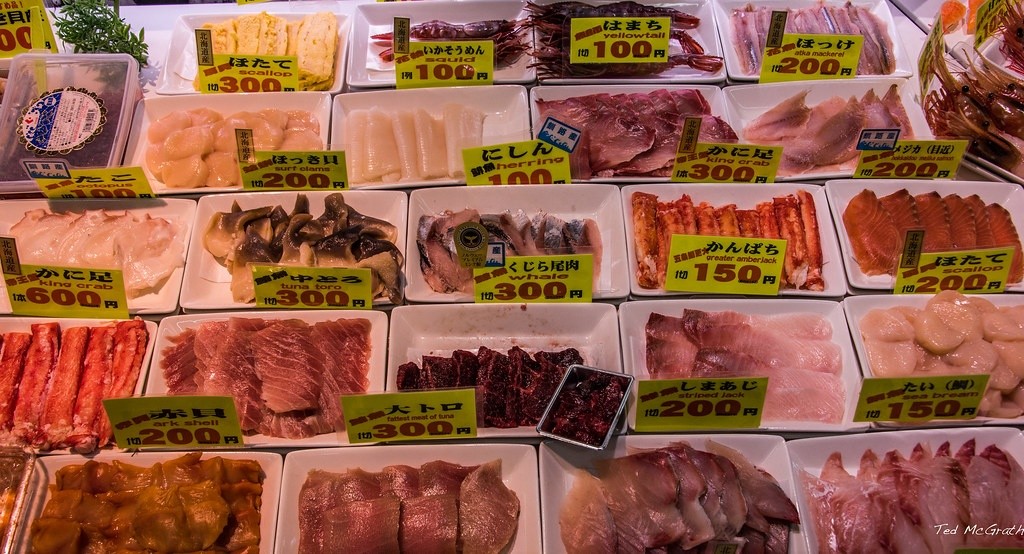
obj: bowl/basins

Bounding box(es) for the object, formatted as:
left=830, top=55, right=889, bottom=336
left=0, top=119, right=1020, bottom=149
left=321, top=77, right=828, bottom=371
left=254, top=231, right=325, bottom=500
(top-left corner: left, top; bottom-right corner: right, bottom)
left=0, top=50, right=141, bottom=197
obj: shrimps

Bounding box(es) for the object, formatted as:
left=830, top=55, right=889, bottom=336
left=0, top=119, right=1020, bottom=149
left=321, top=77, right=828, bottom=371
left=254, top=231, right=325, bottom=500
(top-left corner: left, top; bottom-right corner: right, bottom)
left=369, top=18, right=533, bottom=72
left=927, top=62, right=1024, bottom=181
left=522, top=0, right=724, bottom=81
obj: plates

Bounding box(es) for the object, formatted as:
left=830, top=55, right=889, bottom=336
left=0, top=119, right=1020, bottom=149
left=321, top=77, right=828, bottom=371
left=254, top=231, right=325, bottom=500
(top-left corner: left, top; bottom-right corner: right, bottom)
left=18, top=451, right=282, bottom=554
left=539, top=436, right=807, bottom=554
left=0, top=445, right=36, bottom=554
left=119, top=92, right=331, bottom=195
left=155, top=12, right=351, bottom=93
left=537, top=365, right=635, bottom=451
left=823, top=179, right=1024, bottom=427
left=332, top=85, right=530, bottom=189
left=387, top=184, right=869, bottom=435
left=529, top=85, right=730, bottom=184
left=786, top=427, right=1024, bottom=554
left=0, top=199, right=203, bottom=456
left=722, top=78, right=934, bottom=184
left=273, top=445, right=543, bottom=554
left=344, top=3, right=912, bottom=86
left=146, top=190, right=410, bottom=451
left=927, top=14, right=1024, bottom=185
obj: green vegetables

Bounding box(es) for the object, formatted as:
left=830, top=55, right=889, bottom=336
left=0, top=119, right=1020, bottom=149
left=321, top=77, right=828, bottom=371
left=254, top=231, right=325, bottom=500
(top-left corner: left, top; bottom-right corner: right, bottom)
left=49, top=0, right=149, bottom=73
